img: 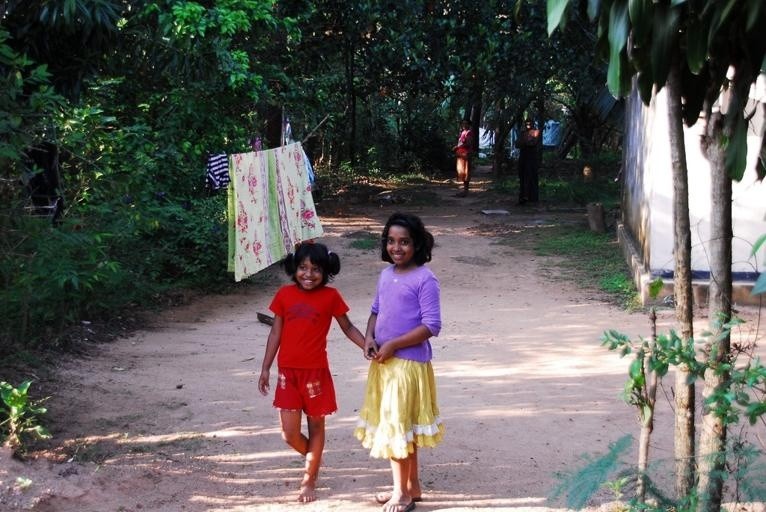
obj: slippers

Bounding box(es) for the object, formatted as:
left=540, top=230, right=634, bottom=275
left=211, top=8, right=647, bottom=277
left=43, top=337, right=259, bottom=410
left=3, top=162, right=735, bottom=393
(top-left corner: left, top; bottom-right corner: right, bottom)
left=374, top=490, right=422, bottom=511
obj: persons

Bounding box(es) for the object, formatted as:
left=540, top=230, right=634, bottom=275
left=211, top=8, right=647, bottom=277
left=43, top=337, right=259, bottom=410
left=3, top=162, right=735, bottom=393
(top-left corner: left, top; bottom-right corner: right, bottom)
left=451, top=119, right=476, bottom=197
left=513, top=118, right=542, bottom=207
left=257, top=241, right=378, bottom=504
left=352, top=213, right=447, bottom=512
